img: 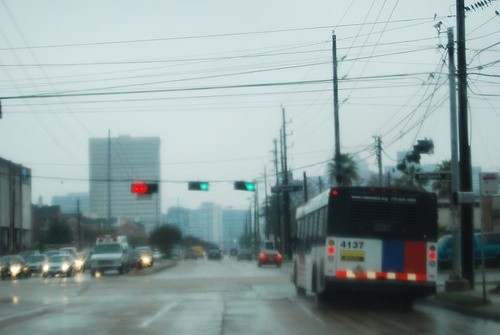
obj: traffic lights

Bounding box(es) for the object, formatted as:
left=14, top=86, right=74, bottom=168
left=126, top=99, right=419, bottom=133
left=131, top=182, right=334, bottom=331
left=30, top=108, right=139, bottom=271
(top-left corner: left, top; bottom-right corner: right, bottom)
left=131, top=183, right=158, bottom=194
left=234, top=180, right=255, bottom=191
left=188, top=182, right=209, bottom=191
left=406, top=150, right=421, bottom=163
left=413, top=139, right=435, bottom=155
left=397, top=162, right=407, bottom=172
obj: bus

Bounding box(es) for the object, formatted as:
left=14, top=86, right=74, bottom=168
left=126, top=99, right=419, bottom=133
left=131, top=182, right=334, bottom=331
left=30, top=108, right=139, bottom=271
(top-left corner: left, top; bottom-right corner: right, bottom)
left=292, top=185, right=438, bottom=309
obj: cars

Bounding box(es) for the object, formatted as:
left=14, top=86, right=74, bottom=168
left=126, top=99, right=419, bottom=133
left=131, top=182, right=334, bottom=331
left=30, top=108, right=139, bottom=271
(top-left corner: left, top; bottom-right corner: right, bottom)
left=131, top=243, right=237, bottom=269
left=258, top=248, right=282, bottom=267
left=1, top=245, right=89, bottom=279
left=237, top=250, right=252, bottom=261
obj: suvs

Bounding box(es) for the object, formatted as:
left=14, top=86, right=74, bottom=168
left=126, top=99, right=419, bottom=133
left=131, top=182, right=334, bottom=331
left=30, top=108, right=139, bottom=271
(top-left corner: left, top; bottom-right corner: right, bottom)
left=90, top=243, right=130, bottom=277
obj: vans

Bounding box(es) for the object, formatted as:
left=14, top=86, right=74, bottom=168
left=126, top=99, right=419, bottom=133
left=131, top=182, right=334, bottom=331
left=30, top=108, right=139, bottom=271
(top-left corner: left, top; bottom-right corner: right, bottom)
left=435, top=232, right=500, bottom=267
left=95, top=233, right=129, bottom=246
